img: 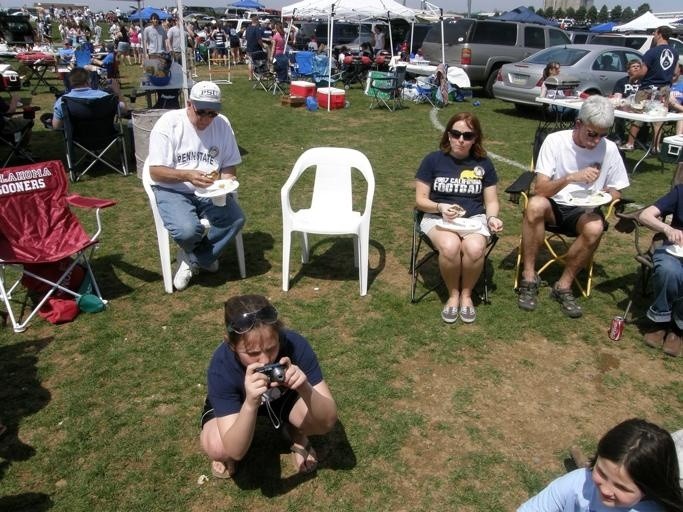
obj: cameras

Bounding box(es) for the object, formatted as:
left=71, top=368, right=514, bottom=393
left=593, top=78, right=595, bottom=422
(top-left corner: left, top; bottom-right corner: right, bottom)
left=254, top=362, right=288, bottom=383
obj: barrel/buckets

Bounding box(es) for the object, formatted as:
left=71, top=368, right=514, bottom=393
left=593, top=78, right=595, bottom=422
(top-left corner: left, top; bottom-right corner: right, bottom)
left=131, top=108, right=172, bottom=180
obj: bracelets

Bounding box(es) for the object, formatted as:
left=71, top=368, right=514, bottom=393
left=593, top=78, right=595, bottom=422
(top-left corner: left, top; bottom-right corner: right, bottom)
left=486, top=216, right=495, bottom=225
left=436, top=203, right=440, bottom=213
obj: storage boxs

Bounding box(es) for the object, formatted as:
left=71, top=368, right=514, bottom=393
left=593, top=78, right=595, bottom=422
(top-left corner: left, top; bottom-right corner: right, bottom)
left=658, top=134, right=683, bottom=164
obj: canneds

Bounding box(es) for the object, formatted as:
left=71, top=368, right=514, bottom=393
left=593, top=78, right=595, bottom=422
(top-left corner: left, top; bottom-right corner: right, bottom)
left=608, top=316, right=625, bottom=340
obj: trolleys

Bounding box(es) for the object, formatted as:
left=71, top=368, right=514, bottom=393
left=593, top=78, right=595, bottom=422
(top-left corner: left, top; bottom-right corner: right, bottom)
left=338, top=53, right=372, bottom=91
left=16, top=51, right=57, bottom=94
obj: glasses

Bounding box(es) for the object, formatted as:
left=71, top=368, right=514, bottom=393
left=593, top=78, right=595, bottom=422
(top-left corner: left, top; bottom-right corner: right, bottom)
left=226, top=305, right=278, bottom=335
left=586, top=128, right=608, bottom=139
left=193, top=106, right=217, bottom=118
left=449, top=129, right=475, bottom=141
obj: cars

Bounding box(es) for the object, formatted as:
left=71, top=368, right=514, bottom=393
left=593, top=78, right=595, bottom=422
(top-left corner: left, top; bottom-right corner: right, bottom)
left=491, top=43, right=643, bottom=123
left=0, top=4, right=62, bottom=46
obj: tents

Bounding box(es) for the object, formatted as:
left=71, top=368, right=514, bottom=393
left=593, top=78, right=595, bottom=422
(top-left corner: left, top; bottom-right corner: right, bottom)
left=281, top=2, right=416, bottom=112
left=227, top=2, right=265, bottom=15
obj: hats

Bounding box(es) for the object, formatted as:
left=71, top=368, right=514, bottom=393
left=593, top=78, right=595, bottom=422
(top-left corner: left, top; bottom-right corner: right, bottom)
left=190, top=81, right=224, bottom=112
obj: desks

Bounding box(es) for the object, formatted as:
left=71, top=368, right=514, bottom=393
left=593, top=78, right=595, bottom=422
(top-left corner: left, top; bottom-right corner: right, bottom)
left=535, top=96, right=683, bottom=176
left=0, top=40, right=193, bottom=183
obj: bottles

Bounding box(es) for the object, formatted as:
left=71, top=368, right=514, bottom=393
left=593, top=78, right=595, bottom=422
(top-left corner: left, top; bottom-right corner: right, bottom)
left=409, top=53, right=430, bottom=66
left=12, top=42, right=54, bottom=54
left=542, top=77, right=667, bottom=117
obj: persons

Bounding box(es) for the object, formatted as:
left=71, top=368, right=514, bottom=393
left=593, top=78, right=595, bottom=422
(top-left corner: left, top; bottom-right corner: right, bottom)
left=414, top=112, right=504, bottom=321
left=518, top=97, right=630, bottom=318
left=570, top=428, right=682, bottom=487
left=540, top=61, right=587, bottom=112
left=201, top=295, right=339, bottom=477
left=189, top=15, right=410, bottom=91
left=638, top=182, right=683, bottom=357
left=613, top=26, right=683, bottom=152
left=515, top=419, right=682, bottom=512
left=146, top=80, right=246, bottom=290
left=1, top=2, right=195, bottom=162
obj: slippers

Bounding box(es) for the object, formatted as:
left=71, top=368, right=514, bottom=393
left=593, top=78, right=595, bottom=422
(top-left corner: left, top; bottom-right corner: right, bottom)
left=212, top=459, right=236, bottom=478
left=291, top=440, right=318, bottom=475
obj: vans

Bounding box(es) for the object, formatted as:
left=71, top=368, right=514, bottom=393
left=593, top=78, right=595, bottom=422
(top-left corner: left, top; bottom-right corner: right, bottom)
left=418, top=19, right=572, bottom=98
left=179, top=6, right=683, bottom=69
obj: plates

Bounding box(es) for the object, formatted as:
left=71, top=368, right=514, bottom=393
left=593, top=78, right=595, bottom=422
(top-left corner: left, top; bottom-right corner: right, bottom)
left=437, top=218, right=481, bottom=231
left=195, top=180, right=239, bottom=198
left=570, top=190, right=612, bottom=206
left=665, top=244, right=683, bottom=259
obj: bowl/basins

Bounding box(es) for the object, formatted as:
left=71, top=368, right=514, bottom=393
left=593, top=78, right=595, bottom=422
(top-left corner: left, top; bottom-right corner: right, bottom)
left=148, top=77, right=169, bottom=85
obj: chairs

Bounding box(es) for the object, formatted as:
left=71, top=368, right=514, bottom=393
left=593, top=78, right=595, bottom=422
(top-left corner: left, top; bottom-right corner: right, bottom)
left=142, top=155, right=246, bottom=293
left=0, top=160, right=117, bottom=333
left=281, top=147, right=375, bottom=296
left=616, top=162, right=683, bottom=322
left=514, top=154, right=620, bottom=299
left=252, top=49, right=484, bottom=113
left=408, top=206, right=499, bottom=304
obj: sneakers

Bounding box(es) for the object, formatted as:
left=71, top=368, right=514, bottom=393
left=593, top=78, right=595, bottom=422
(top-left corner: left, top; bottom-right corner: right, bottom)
left=550, top=282, right=582, bottom=318
left=663, top=332, right=682, bottom=356
left=518, top=271, right=540, bottom=310
left=459, top=306, right=476, bottom=322
left=644, top=327, right=665, bottom=347
left=619, top=144, right=634, bottom=151
left=178, top=250, right=219, bottom=272
left=173, top=261, right=193, bottom=291
left=441, top=306, right=458, bottom=323
